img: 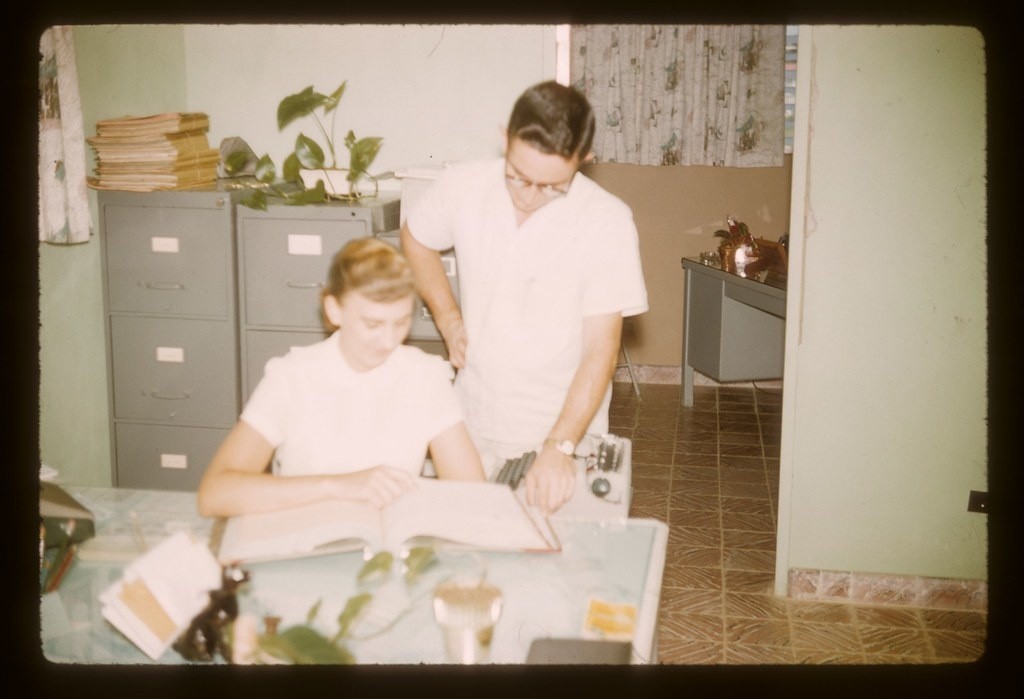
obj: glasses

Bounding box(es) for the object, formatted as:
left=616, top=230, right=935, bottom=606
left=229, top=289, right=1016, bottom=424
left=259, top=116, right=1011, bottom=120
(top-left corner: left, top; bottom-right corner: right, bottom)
left=503, top=139, right=579, bottom=198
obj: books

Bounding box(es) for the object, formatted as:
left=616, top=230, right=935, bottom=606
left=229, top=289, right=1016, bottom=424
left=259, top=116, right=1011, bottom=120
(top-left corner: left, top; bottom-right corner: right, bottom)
left=86, top=110, right=221, bottom=193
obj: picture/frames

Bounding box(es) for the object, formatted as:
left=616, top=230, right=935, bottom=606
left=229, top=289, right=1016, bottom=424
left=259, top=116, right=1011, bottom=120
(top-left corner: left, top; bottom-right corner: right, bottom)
left=755, top=238, right=788, bottom=273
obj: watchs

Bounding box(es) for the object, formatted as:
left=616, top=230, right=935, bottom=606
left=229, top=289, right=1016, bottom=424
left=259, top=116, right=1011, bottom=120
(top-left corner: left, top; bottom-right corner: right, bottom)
left=543, top=438, right=575, bottom=456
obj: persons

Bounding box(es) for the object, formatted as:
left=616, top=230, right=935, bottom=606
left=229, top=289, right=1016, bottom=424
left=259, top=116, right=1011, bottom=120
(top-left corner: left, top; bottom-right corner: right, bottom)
left=400, top=79, right=650, bottom=516
left=196, top=238, right=486, bottom=518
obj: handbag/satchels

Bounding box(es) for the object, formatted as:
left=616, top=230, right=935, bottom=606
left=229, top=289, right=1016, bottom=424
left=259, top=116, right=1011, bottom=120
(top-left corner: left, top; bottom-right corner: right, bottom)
left=40, top=479, right=95, bottom=594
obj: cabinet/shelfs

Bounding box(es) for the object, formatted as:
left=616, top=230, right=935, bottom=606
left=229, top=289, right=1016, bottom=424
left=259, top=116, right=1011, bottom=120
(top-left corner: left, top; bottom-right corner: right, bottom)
left=99, top=175, right=275, bottom=488
left=236, top=192, right=400, bottom=410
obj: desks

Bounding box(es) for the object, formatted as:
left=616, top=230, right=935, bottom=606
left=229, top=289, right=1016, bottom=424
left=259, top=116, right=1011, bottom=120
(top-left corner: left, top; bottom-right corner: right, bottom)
left=680, top=257, right=786, bottom=409
left=42, top=485, right=673, bottom=665
